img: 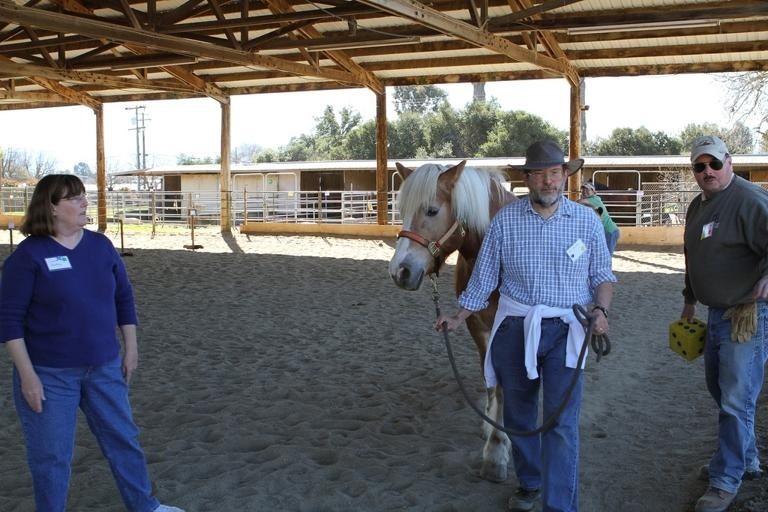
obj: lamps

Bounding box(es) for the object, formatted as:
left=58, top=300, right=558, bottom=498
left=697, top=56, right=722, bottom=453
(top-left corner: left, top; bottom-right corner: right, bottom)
left=567, top=18, right=720, bottom=35
left=109, top=57, right=198, bottom=72
left=307, top=36, right=420, bottom=51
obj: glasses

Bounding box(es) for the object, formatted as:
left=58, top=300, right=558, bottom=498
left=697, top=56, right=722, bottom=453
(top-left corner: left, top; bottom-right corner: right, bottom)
left=61, top=195, right=87, bottom=201
left=693, top=161, right=723, bottom=172
left=528, top=170, right=562, bottom=176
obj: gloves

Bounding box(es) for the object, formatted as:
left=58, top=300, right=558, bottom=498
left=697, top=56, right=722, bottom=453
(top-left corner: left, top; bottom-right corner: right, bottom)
left=722, top=302, right=757, bottom=343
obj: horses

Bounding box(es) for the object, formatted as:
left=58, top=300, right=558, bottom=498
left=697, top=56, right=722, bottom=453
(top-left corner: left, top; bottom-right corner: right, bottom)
left=389, top=160, right=523, bottom=483
left=588, top=179, right=636, bottom=226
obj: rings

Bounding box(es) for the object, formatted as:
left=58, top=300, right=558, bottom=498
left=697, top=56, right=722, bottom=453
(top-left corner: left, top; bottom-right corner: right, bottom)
left=599, top=328, right=606, bottom=334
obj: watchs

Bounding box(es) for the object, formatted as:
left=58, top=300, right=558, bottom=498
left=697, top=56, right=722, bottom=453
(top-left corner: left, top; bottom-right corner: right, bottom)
left=592, top=306, right=609, bottom=318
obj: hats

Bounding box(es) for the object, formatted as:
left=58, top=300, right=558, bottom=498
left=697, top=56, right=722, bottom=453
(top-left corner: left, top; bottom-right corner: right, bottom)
left=581, top=182, right=595, bottom=190
left=690, top=135, right=729, bottom=161
left=507, top=141, right=584, bottom=175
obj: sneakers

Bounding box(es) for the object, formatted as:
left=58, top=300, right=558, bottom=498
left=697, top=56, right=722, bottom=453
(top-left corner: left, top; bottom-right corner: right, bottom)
left=509, top=486, right=542, bottom=510
left=696, top=488, right=737, bottom=511
left=155, top=504, right=185, bottom=512
left=700, top=465, right=762, bottom=481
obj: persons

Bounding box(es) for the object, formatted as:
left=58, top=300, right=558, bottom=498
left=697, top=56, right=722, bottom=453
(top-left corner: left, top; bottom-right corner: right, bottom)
left=576, top=182, right=619, bottom=258
left=680, top=135, right=767, bottom=512
left=431, top=141, right=617, bottom=511
left=0, top=173, right=186, bottom=511
